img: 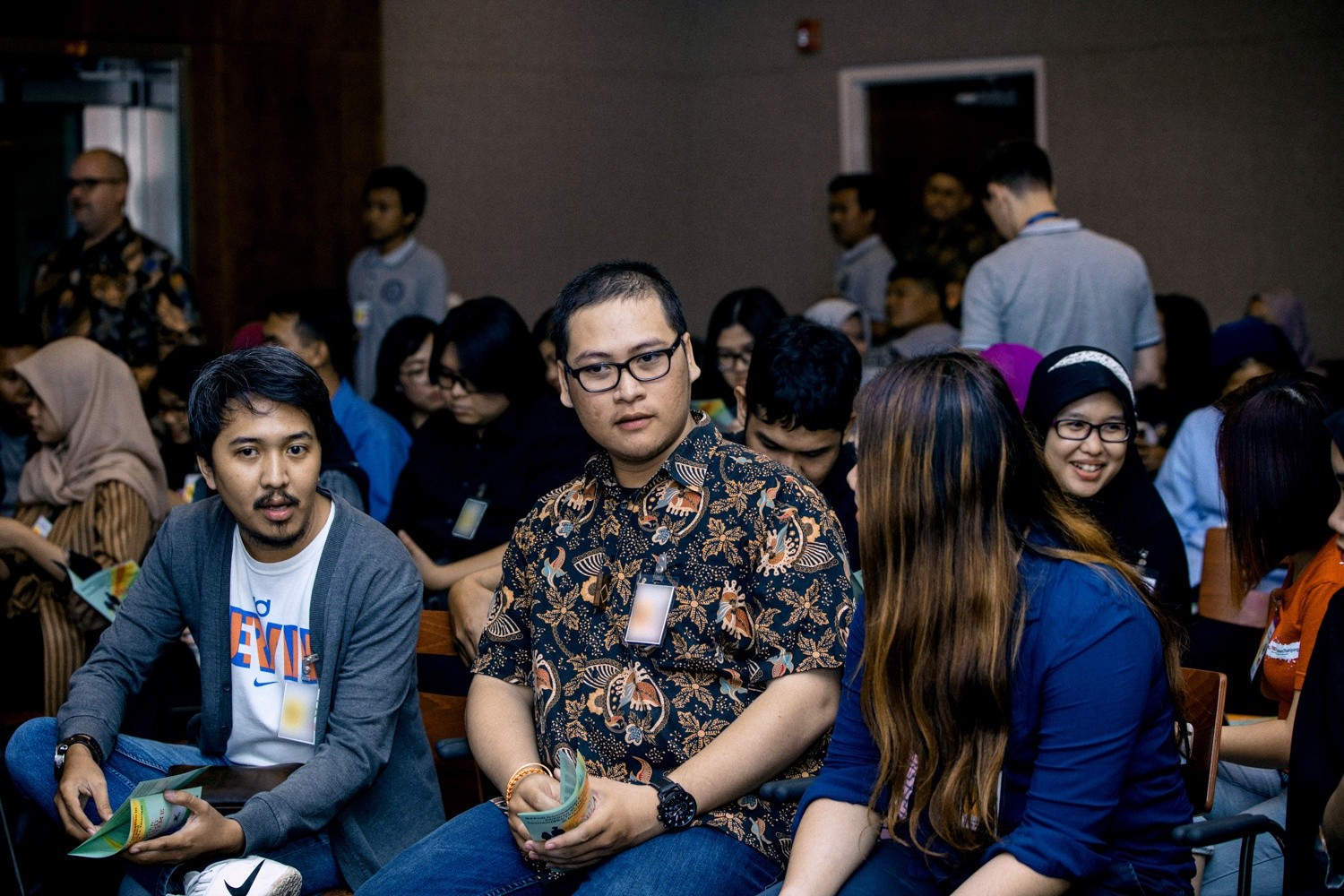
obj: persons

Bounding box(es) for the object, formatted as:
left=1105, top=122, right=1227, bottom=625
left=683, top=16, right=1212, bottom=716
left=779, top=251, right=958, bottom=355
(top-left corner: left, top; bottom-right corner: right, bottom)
left=1174, top=370, right=1344, bottom=896
left=6, top=348, right=446, bottom=896
left=798, top=296, right=889, bottom=385
left=530, top=304, right=563, bottom=391
left=828, top=143, right=1163, bottom=412
left=758, top=355, right=1198, bottom=896
left=705, top=286, right=784, bottom=446
left=347, top=166, right=446, bottom=402
left=1154, top=318, right=1304, bottom=616
left=355, top=260, right=854, bottom=896
left=1242, top=290, right=1310, bottom=355
left=368, top=313, right=447, bottom=433
left=1021, top=344, right=1192, bottom=640
left=260, top=292, right=413, bottom=524
left=385, top=296, right=607, bottom=696
left=1146, top=292, right=1210, bottom=444
left=446, top=315, right=860, bottom=670
left=1, top=335, right=169, bottom=749
left=26, top=148, right=201, bottom=488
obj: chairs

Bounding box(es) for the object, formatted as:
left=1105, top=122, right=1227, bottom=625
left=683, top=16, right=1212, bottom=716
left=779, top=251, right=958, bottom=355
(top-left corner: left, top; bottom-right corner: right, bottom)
left=419, top=447, right=1300, bottom=896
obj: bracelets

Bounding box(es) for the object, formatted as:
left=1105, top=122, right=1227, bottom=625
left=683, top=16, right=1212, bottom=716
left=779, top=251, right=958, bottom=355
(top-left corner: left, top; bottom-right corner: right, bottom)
left=507, top=768, right=547, bottom=805
left=508, top=762, right=553, bottom=788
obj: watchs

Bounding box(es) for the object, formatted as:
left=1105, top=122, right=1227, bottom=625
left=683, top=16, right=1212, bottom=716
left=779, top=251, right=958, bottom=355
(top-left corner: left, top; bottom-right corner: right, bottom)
left=648, top=776, right=697, bottom=831
left=52, top=734, right=105, bottom=780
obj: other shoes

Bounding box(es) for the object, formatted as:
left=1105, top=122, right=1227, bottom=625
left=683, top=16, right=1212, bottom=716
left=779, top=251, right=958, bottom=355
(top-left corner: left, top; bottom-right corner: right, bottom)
left=165, top=854, right=302, bottom=896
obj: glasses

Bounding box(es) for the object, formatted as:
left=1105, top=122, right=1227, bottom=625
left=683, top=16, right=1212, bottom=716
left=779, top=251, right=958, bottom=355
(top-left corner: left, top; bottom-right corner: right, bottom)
left=436, top=369, right=483, bottom=394
left=64, top=174, right=124, bottom=194
left=1054, top=418, right=1131, bottom=443
left=409, top=370, right=428, bottom=387
left=563, top=334, right=681, bottom=393
left=716, top=348, right=750, bottom=373
